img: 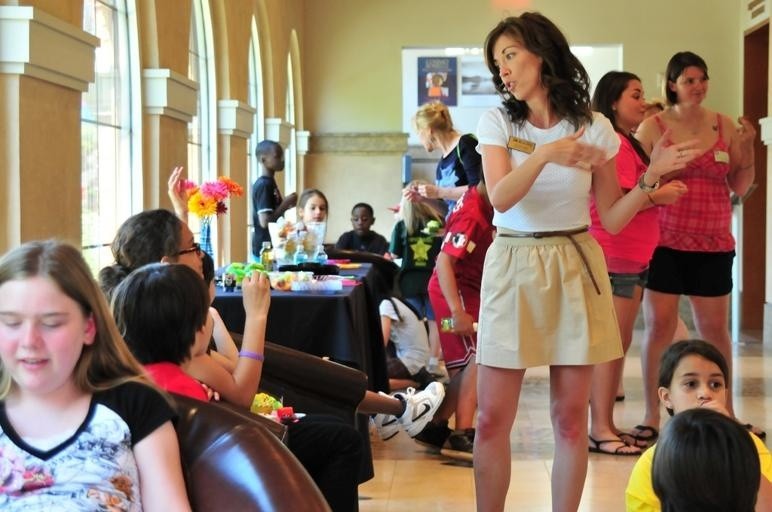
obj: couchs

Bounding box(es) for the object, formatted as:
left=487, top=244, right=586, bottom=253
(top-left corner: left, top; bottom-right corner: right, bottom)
left=207, top=332, right=375, bottom=512
left=163, top=391, right=333, bottom=511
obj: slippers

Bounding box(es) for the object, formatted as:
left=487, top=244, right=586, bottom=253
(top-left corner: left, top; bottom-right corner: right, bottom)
left=741, top=422, right=766, bottom=438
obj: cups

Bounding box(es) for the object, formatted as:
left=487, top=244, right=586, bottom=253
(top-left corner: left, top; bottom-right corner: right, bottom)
left=305, top=220, right=327, bottom=241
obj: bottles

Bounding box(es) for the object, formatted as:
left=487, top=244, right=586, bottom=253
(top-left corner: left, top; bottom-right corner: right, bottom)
left=261, top=240, right=328, bottom=272
left=440, top=317, right=478, bottom=334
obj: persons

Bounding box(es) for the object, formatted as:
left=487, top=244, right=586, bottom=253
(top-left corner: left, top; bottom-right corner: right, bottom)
left=472, top=13, right=703, bottom=512
left=379, top=51, right=772, bottom=512
left=1, top=138, right=448, bottom=511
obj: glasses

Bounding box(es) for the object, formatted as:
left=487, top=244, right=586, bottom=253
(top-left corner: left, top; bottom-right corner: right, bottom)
left=169, top=243, right=200, bottom=258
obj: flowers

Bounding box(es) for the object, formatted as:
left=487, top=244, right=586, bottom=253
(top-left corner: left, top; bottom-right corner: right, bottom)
left=176, top=177, right=244, bottom=218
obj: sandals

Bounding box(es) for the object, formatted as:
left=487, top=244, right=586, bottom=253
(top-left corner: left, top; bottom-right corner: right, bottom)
left=589, top=424, right=659, bottom=456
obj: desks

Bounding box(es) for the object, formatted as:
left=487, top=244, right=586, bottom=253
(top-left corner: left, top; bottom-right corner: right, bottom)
left=210, top=262, right=390, bottom=419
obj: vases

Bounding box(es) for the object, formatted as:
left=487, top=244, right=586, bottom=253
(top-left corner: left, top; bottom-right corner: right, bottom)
left=197, top=218, right=214, bottom=258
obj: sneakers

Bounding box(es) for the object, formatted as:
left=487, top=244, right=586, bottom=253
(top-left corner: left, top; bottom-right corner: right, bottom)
left=366, top=365, right=476, bottom=464
